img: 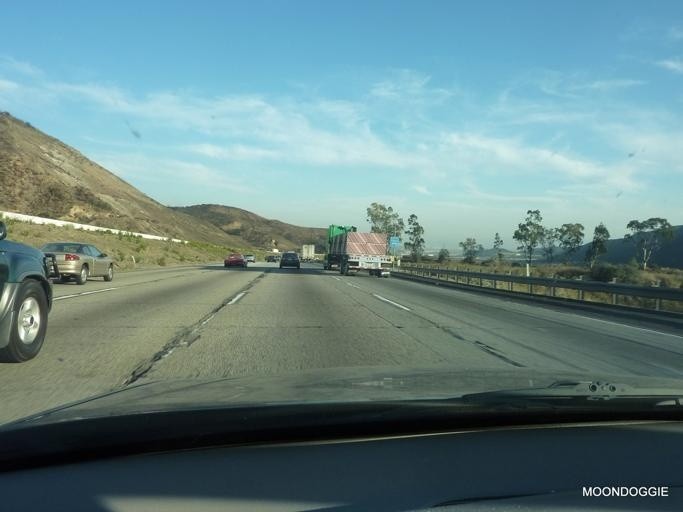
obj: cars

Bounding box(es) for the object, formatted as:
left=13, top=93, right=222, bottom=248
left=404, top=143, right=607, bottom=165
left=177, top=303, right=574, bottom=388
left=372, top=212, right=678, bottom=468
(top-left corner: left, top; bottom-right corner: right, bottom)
left=369, top=269, right=391, bottom=277
left=264, top=254, right=281, bottom=262
left=322, top=254, right=327, bottom=270
left=244, top=254, right=255, bottom=262
left=224, top=253, right=248, bottom=268
left=38, top=240, right=114, bottom=282
left=279, top=252, right=300, bottom=269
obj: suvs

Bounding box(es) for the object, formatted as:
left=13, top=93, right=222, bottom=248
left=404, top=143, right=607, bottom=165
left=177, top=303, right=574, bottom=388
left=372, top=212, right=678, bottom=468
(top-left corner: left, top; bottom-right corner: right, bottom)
left=1, top=220, right=54, bottom=366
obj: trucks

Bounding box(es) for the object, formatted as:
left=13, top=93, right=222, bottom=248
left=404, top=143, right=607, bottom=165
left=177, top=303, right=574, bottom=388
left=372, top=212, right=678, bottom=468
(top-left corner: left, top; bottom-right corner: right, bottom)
left=325, top=225, right=393, bottom=276
